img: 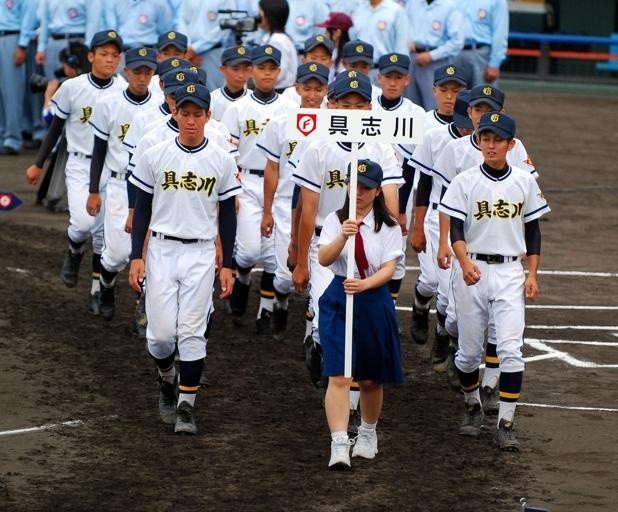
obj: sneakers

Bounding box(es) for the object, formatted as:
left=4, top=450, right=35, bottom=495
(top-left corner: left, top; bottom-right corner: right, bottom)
left=0, top=147, right=19, bottom=155
left=25, top=140, right=42, bottom=150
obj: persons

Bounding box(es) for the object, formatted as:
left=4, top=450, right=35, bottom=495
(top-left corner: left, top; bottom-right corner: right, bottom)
left=128, top=81, right=243, bottom=436
left=430, top=86, right=541, bottom=409
left=0, top=0, right=510, bottom=341
left=125, top=70, right=241, bottom=389
left=316, top=159, right=406, bottom=469
left=406, top=89, right=476, bottom=364
left=436, top=111, right=551, bottom=449
left=290, top=79, right=407, bottom=433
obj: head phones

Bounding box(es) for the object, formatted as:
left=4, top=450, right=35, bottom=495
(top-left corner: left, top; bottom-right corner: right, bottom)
left=64, top=40, right=80, bottom=68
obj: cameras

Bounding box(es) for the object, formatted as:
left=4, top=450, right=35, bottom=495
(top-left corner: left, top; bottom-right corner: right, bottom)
left=218, top=9, right=262, bottom=31
left=30, top=68, right=66, bottom=94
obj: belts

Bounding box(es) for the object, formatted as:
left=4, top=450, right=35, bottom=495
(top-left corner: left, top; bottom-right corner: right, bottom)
left=67, top=151, right=91, bottom=158
left=1, top=30, right=19, bottom=36
left=152, top=231, right=197, bottom=244
left=52, top=33, right=84, bottom=40
left=315, top=227, right=321, bottom=236
left=433, top=203, right=438, bottom=209
